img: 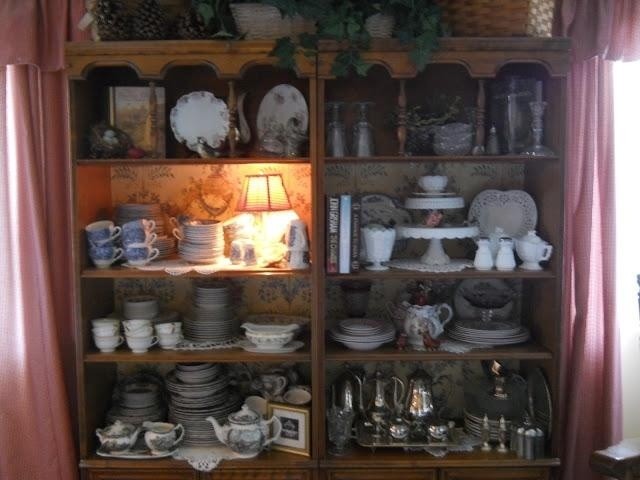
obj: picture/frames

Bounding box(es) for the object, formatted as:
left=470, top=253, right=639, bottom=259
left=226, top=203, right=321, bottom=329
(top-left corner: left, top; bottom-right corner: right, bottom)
left=264, top=399, right=311, bottom=459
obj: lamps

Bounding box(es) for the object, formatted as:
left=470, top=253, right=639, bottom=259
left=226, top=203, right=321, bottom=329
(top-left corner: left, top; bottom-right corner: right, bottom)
left=236, top=172, right=294, bottom=269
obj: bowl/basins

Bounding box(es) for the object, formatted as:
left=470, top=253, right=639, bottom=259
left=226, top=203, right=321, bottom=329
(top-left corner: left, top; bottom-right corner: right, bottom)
left=240, top=319, right=299, bottom=349
left=431, top=124, right=473, bottom=155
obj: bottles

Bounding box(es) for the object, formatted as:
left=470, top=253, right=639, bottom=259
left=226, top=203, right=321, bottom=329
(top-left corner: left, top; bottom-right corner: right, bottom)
left=488, top=126, right=501, bottom=154
left=230, top=241, right=242, bottom=264
left=353, top=106, right=376, bottom=157
left=496, top=233, right=515, bottom=270
left=474, top=237, right=493, bottom=270
left=243, top=243, right=256, bottom=266
left=327, top=101, right=349, bottom=158
left=480, top=410, right=545, bottom=461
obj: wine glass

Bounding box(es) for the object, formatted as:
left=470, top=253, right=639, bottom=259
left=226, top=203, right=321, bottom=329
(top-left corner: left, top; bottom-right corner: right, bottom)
left=361, top=229, right=396, bottom=271
left=455, top=288, right=521, bottom=329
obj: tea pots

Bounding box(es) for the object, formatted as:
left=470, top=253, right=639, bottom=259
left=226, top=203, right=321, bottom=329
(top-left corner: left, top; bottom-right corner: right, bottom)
left=206, top=404, right=283, bottom=459
left=326, top=365, right=460, bottom=456
left=515, top=230, right=553, bottom=270
left=94, top=419, right=140, bottom=454
left=143, top=420, right=184, bottom=454
left=387, top=297, right=453, bottom=347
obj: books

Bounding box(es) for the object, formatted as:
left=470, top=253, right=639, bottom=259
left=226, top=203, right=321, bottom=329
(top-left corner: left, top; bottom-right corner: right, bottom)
left=323, top=196, right=362, bottom=275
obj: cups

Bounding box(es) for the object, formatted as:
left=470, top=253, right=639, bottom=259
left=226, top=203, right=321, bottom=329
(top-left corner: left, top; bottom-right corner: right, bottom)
left=254, top=369, right=311, bottom=406
left=417, top=176, right=447, bottom=192
left=92, top=296, right=183, bottom=354
left=83, top=219, right=159, bottom=268
left=284, top=220, right=311, bottom=269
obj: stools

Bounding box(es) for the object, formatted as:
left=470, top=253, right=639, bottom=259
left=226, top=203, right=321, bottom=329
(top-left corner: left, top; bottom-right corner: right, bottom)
left=589, top=436, right=639, bottom=480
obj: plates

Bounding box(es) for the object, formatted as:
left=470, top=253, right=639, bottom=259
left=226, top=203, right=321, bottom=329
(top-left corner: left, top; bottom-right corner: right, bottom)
left=448, top=319, right=530, bottom=347
left=329, top=316, right=395, bottom=351
left=468, top=190, right=537, bottom=254
left=463, top=405, right=512, bottom=444
left=526, top=366, right=554, bottom=440
left=169, top=91, right=229, bottom=148
left=411, top=191, right=455, bottom=198
left=183, top=279, right=241, bottom=342
left=115, top=203, right=177, bottom=258
left=256, top=84, right=311, bottom=151
left=94, top=448, right=176, bottom=462
left=358, top=194, right=412, bottom=258
left=238, top=342, right=305, bottom=354
left=106, top=361, right=241, bottom=447
left=175, top=218, right=224, bottom=264
left=452, top=277, right=516, bottom=321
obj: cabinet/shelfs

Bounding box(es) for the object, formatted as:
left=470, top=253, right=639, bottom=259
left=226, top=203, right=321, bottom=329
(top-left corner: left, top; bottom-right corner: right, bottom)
left=64, top=38, right=317, bottom=480
left=318, top=35, right=579, bottom=480
